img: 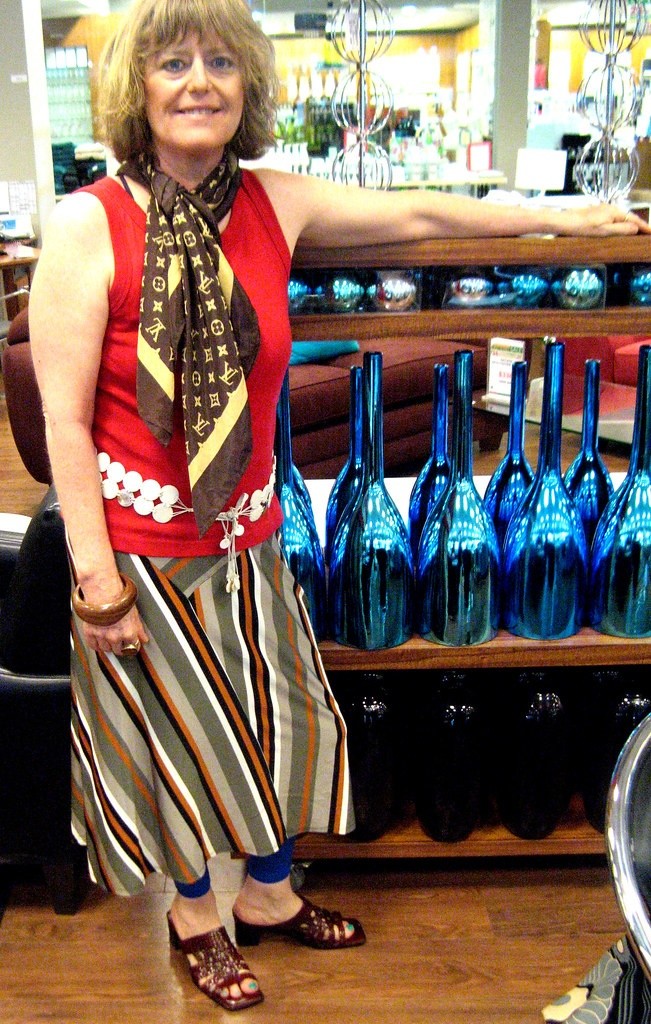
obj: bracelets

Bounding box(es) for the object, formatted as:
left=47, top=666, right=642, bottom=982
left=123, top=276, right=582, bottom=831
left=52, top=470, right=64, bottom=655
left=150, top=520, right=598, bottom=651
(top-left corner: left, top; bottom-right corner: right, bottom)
left=72, top=573, right=138, bottom=626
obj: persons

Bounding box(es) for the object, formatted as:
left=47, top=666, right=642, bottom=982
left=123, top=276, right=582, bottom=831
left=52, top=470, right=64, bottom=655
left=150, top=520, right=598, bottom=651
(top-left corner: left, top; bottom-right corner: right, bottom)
left=29, top=0, right=650, bottom=1011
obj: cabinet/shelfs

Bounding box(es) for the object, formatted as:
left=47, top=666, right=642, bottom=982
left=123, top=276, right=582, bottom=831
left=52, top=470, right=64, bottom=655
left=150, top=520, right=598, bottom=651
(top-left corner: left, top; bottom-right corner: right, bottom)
left=289, top=233, right=651, bottom=890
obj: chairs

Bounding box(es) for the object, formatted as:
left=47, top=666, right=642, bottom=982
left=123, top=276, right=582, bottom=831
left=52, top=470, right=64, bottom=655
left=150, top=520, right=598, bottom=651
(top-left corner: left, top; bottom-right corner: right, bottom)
left=0, top=509, right=87, bottom=916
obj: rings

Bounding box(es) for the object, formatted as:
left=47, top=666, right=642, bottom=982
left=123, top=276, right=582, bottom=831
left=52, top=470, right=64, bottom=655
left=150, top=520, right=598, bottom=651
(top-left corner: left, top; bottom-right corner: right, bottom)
left=121, top=639, right=139, bottom=655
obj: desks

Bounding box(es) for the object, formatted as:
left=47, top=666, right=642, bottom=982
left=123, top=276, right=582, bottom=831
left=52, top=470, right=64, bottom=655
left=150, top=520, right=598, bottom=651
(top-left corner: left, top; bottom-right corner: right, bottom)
left=472, top=373, right=636, bottom=444
left=0, top=238, right=40, bottom=321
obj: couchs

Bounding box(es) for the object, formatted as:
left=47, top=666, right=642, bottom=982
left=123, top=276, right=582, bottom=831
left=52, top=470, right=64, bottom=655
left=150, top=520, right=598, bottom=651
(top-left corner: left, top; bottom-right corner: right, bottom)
left=2, top=307, right=507, bottom=486
left=555, top=335, right=651, bottom=385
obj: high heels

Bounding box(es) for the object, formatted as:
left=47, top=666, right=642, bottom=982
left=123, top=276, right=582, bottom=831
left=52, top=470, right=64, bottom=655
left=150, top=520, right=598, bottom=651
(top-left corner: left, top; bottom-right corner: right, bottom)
left=232, top=894, right=366, bottom=950
left=167, top=910, right=264, bottom=1011
